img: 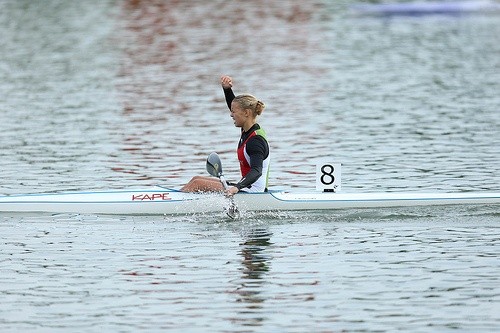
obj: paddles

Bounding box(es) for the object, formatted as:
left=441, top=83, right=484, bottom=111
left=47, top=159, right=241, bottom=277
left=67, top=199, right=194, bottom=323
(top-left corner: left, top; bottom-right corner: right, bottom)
left=206, top=152, right=246, bottom=221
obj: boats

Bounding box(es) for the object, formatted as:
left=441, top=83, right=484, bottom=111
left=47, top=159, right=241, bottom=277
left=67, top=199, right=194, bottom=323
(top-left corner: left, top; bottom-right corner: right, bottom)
left=0, top=186, right=499, bottom=215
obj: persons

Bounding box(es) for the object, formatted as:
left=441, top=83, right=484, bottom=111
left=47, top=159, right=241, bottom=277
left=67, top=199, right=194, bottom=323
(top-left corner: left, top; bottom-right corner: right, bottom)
left=180, top=76, right=270, bottom=198
